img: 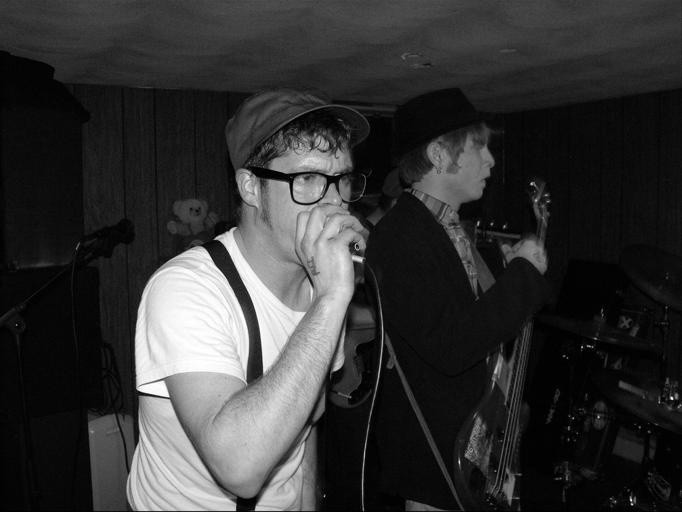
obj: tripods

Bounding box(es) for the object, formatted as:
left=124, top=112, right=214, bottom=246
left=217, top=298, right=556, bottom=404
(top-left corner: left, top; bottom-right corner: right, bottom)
left=553, top=307, right=682, bottom=512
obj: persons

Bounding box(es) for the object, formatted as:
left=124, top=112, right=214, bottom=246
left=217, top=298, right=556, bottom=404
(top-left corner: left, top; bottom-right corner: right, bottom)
left=359, top=83, right=550, bottom=512
left=121, top=86, right=370, bottom=512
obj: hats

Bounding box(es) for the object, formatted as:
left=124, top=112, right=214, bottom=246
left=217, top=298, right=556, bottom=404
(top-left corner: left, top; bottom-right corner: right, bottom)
left=393, top=87, right=486, bottom=156
left=225, top=87, right=371, bottom=173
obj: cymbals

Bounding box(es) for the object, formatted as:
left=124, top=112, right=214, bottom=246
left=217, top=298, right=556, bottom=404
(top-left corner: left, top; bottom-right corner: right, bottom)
left=590, top=325, right=661, bottom=359
left=598, top=376, right=682, bottom=436
left=624, top=264, right=682, bottom=314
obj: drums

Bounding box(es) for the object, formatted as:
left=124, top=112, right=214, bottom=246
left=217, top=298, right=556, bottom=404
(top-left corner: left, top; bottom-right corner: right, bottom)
left=561, top=399, right=656, bottom=510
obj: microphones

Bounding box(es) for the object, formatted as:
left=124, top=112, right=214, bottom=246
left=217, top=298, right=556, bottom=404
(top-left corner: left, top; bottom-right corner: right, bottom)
left=76, top=217, right=137, bottom=245
left=349, top=242, right=366, bottom=285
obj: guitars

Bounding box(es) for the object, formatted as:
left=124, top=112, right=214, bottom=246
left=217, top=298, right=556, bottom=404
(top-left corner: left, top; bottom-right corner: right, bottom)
left=451, top=175, right=552, bottom=512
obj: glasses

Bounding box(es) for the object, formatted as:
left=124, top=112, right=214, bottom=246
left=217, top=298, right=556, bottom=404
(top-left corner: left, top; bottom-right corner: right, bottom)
left=247, top=166, right=367, bottom=205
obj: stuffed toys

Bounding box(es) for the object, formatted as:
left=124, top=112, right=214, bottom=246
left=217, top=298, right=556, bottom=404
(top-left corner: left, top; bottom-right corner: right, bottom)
left=165, top=197, right=221, bottom=251
